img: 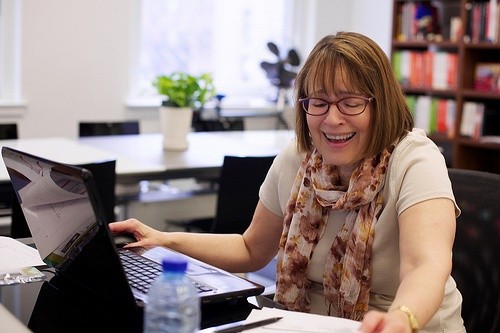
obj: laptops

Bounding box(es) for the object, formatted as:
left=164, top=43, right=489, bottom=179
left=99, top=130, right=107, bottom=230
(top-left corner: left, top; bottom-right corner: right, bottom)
left=1, top=146, right=265, bottom=308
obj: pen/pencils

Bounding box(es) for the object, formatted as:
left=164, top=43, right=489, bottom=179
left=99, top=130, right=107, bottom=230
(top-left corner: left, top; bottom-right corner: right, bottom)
left=215, top=316, right=285, bottom=333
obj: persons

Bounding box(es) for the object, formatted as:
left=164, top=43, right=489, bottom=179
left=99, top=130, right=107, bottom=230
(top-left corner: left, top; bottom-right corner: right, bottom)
left=106, top=32, right=467, bottom=333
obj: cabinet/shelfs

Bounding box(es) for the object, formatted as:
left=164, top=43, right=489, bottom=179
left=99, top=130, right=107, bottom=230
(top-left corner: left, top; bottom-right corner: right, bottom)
left=391, top=0, right=500, bottom=171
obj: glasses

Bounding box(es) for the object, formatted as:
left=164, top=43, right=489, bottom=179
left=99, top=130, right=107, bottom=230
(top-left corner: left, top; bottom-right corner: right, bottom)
left=298, top=94, right=374, bottom=115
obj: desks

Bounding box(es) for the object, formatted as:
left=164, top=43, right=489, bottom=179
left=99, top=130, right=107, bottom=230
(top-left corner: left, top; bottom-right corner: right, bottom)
left=0, top=245, right=287, bottom=333
left=0, top=130, right=295, bottom=198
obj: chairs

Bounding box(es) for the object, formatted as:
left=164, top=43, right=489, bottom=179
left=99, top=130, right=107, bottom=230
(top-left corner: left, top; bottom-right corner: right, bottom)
left=78, top=122, right=140, bottom=219
left=161, top=154, right=276, bottom=235
left=446, top=167, right=500, bottom=333
left=192, top=116, right=244, bottom=194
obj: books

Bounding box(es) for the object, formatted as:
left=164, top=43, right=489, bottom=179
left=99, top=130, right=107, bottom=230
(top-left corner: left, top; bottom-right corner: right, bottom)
left=476, top=64, right=498, bottom=93
left=460, top=103, right=487, bottom=138
left=400, top=96, right=456, bottom=137
left=395, top=0, right=500, bottom=45
left=389, top=50, right=460, bottom=91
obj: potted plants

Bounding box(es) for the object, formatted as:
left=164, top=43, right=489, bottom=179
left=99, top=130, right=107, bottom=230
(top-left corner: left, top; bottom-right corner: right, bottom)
left=154, top=71, right=215, bottom=150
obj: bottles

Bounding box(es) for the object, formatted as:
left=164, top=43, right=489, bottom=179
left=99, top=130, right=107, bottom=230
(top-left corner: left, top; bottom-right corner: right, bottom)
left=144, top=257, right=201, bottom=333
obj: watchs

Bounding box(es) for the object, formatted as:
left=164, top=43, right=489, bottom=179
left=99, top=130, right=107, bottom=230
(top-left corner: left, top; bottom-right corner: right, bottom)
left=389, top=305, right=418, bottom=333
